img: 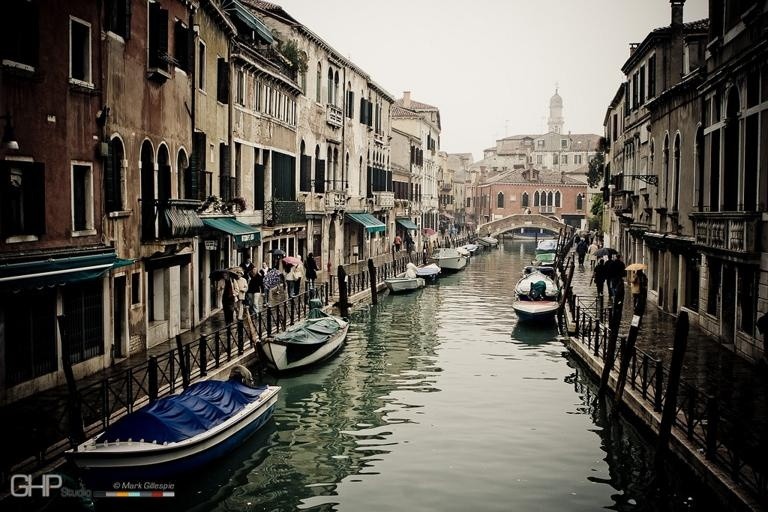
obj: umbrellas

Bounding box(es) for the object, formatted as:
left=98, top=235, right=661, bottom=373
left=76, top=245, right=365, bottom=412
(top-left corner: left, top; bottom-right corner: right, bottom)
left=420, top=227, right=434, bottom=235
left=208, top=268, right=238, bottom=281
left=281, top=256, right=302, bottom=266
left=623, top=262, right=648, bottom=271
left=266, top=248, right=286, bottom=257
left=591, top=246, right=617, bottom=256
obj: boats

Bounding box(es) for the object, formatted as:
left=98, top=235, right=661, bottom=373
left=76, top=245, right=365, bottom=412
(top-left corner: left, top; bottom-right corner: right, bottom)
left=385, top=235, right=499, bottom=295
left=256, top=298, right=350, bottom=378
left=511, top=240, right=561, bottom=328
left=64, top=364, right=281, bottom=506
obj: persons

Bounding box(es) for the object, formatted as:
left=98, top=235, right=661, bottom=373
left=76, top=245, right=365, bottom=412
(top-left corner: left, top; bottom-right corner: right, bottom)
left=636, top=270, right=648, bottom=308
left=440, top=221, right=475, bottom=239
left=592, top=254, right=627, bottom=300
left=573, top=234, right=590, bottom=246
left=393, top=231, right=401, bottom=252
left=402, top=231, right=414, bottom=253
left=303, top=253, right=319, bottom=289
left=248, top=267, right=264, bottom=318
left=587, top=241, right=598, bottom=266
left=421, top=229, right=429, bottom=248
left=233, top=269, right=248, bottom=321
left=292, top=263, right=303, bottom=295
left=575, top=238, right=587, bottom=265
left=237, top=256, right=273, bottom=284
left=630, top=272, right=639, bottom=310
left=215, top=272, right=239, bottom=327
left=282, top=262, right=295, bottom=299
